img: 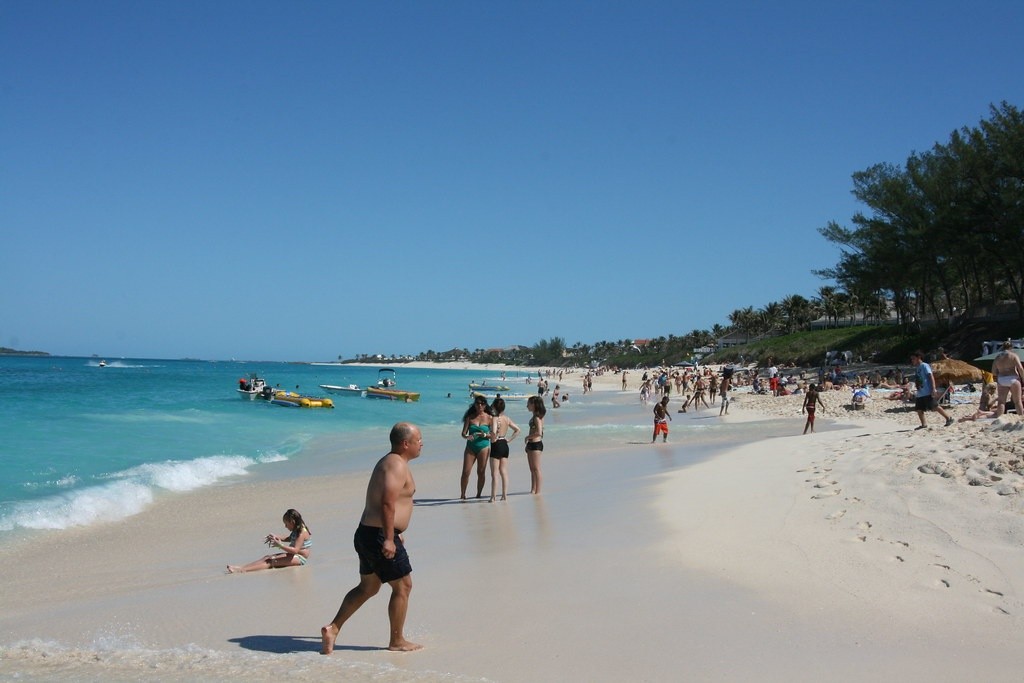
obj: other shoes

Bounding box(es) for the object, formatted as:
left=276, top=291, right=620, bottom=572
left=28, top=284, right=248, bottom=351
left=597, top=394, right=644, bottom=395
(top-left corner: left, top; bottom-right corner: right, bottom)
left=944, top=416, right=954, bottom=426
left=915, top=426, right=927, bottom=430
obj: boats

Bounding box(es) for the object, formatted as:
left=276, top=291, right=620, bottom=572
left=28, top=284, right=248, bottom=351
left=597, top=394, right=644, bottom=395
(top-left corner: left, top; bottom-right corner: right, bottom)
left=234, top=378, right=267, bottom=402
left=263, top=386, right=337, bottom=410
left=99, top=361, right=106, bottom=366
left=375, top=368, right=397, bottom=389
left=470, top=391, right=539, bottom=400
left=318, top=385, right=368, bottom=398
left=366, top=387, right=421, bottom=402
left=469, top=383, right=510, bottom=391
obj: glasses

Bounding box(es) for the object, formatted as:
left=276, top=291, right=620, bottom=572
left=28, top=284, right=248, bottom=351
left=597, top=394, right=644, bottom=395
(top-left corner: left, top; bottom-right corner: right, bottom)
left=474, top=401, right=486, bottom=405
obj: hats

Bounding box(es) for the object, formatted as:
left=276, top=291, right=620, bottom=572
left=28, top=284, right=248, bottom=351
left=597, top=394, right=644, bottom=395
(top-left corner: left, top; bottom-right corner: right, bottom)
left=555, top=386, right=560, bottom=389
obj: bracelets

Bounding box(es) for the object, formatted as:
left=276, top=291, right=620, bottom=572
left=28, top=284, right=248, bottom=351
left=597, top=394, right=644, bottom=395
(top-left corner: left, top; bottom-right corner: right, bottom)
left=482, top=433, right=487, bottom=438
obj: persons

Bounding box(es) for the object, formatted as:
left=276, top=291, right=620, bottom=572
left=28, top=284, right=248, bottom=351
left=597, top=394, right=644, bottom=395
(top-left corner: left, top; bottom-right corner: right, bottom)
left=277, top=383, right=300, bottom=389
left=910, top=346, right=954, bottom=430
left=461, top=396, right=520, bottom=503
left=226, top=509, right=311, bottom=573
left=525, top=396, right=546, bottom=494
left=321, top=422, right=424, bottom=654
left=972, top=340, right=1024, bottom=420
left=653, top=396, right=672, bottom=442
left=447, top=351, right=910, bottom=434
